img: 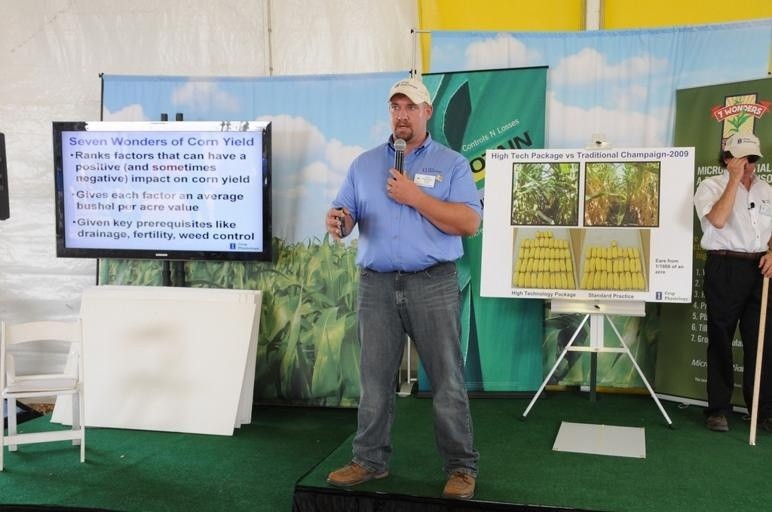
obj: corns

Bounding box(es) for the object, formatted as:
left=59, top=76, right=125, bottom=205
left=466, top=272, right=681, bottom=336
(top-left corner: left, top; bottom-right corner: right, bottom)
left=513, top=231, right=575, bottom=289
left=580, top=239, right=645, bottom=290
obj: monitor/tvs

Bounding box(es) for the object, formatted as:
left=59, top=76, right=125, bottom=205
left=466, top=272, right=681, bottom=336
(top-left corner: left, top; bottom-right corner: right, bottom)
left=52, top=120, right=273, bottom=263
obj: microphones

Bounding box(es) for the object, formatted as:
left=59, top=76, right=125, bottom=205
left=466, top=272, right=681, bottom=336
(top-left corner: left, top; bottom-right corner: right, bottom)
left=393, top=138, right=406, bottom=180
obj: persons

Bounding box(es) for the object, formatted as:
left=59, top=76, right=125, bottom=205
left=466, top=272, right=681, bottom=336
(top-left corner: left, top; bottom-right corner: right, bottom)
left=693, top=133, right=770, bottom=432
left=326, top=79, right=483, bottom=499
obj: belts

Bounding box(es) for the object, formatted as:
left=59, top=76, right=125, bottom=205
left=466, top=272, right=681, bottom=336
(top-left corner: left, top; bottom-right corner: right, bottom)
left=711, top=249, right=764, bottom=261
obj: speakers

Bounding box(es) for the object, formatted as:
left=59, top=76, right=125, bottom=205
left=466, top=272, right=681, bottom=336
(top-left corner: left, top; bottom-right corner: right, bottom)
left=0, top=132, right=10, bottom=221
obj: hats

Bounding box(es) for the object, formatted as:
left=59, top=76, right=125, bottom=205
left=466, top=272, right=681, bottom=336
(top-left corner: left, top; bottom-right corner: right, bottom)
left=723, top=131, right=764, bottom=158
left=388, top=78, right=430, bottom=108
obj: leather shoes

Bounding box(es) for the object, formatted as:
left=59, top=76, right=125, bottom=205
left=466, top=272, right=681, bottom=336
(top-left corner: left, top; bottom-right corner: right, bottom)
left=440, top=469, right=476, bottom=501
left=758, top=415, right=772, bottom=432
left=326, top=461, right=390, bottom=488
left=706, top=415, right=729, bottom=431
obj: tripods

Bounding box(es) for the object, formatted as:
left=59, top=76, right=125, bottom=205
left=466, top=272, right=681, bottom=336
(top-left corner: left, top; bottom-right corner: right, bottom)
left=518, top=300, right=676, bottom=430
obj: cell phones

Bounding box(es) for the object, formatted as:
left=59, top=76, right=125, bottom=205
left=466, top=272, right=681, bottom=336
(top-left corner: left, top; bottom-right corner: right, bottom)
left=335, top=208, right=347, bottom=239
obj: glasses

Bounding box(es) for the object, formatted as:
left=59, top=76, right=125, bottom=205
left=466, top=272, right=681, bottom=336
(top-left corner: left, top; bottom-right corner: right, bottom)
left=748, top=156, right=758, bottom=164
left=388, top=104, right=417, bottom=112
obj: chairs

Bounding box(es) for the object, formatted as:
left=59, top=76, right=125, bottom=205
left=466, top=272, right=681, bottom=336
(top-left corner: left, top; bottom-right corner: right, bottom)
left=1, top=317, right=87, bottom=471
